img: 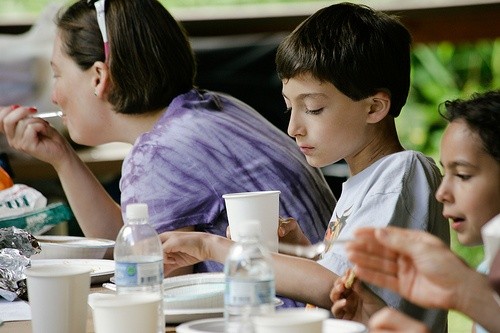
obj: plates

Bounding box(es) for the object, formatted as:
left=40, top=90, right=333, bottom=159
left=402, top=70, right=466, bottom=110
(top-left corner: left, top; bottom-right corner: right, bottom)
left=153, top=295, right=284, bottom=323
left=175, top=316, right=367, bottom=333
left=30, top=260, right=116, bottom=284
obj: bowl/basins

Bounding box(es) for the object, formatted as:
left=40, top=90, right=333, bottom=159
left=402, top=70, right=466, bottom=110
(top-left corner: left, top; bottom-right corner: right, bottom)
left=249, top=308, right=330, bottom=333
left=163, top=272, right=226, bottom=307
left=33, top=235, right=116, bottom=259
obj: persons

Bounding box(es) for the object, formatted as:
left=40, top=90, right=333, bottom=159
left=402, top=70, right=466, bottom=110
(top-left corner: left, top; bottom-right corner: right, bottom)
left=134, top=2, right=450, bottom=333
left=0, top=0, right=339, bottom=308
left=327, top=89, right=500, bottom=333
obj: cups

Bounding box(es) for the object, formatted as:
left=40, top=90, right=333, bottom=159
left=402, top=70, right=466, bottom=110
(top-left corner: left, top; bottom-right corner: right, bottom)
left=87, top=291, right=163, bottom=333
left=222, top=190, right=281, bottom=252
left=24, top=263, right=94, bottom=333
left=224, top=220, right=276, bottom=306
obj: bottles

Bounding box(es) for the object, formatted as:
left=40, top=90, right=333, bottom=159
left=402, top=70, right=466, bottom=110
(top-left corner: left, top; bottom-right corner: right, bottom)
left=113, top=203, right=164, bottom=292
